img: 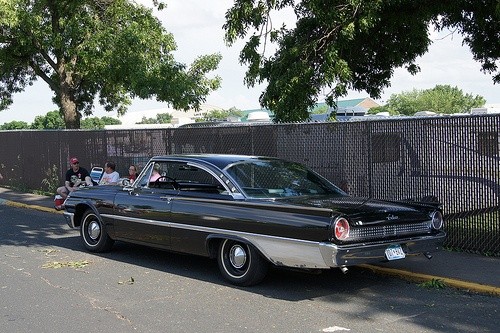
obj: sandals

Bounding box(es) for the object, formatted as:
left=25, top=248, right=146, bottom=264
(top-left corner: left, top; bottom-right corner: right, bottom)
left=54, top=204, right=66, bottom=211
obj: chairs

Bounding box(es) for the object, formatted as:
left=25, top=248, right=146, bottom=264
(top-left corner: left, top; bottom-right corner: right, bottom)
left=90, top=167, right=103, bottom=184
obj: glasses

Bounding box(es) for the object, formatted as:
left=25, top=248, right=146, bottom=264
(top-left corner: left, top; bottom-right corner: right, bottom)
left=128, top=169, right=133, bottom=171
left=73, top=162, right=80, bottom=165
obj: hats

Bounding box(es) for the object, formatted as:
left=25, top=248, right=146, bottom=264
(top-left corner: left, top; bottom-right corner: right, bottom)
left=69, top=156, right=80, bottom=164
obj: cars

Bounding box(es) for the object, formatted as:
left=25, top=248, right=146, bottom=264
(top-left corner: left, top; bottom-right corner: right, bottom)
left=59, top=152, right=448, bottom=288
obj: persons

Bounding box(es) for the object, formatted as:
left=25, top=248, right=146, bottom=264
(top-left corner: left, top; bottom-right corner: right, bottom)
left=149, top=163, right=163, bottom=182
left=98, top=162, right=120, bottom=185
left=54, top=157, right=93, bottom=211
left=123, top=165, right=139, bottom=184
